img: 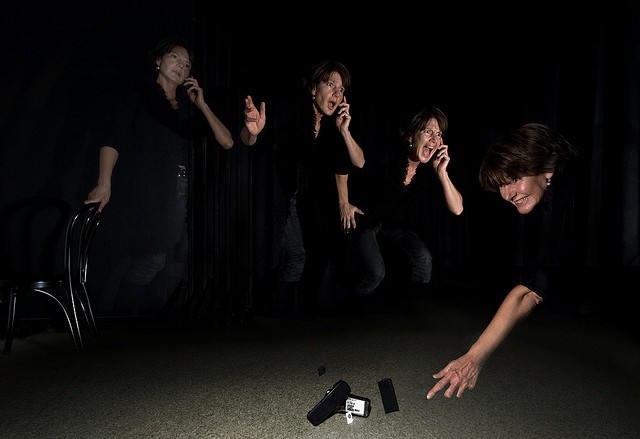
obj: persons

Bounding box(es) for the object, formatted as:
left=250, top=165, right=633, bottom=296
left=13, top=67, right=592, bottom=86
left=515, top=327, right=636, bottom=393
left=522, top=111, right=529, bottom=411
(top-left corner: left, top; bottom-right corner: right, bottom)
left=425, top=122, right=581, bottom=401
left=237, top=59, right=365, bottom=313
left=79, top=38, right=233, bottom=319
left=330, top=109, right=463, bottom=315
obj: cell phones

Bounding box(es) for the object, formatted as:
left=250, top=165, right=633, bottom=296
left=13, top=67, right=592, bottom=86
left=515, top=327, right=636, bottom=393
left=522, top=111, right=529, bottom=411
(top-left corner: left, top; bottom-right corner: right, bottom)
left=337, top=396, right=369, bottom=416
left=307, top=380, right=350, bottom=425
left=378, top=378, right=400, bottom=413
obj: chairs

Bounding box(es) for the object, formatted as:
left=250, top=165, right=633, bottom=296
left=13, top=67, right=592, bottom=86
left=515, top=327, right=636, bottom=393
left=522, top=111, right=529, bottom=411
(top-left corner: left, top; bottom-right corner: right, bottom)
left=4, top=200, right=104, bottom=356
